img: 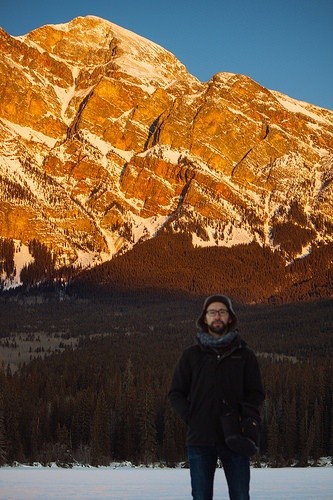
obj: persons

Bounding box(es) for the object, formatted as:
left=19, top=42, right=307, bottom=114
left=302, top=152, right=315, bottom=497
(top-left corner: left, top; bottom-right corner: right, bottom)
left=165, top=292, right=266, bottom=500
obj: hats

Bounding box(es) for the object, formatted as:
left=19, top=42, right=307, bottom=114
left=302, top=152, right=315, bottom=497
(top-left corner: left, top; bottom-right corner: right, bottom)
left=197, top=295, right=238, bottom=330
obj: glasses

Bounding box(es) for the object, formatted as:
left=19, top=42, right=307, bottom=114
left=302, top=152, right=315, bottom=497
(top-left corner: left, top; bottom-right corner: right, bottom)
left=206, top=310, right=229, bottom=316
